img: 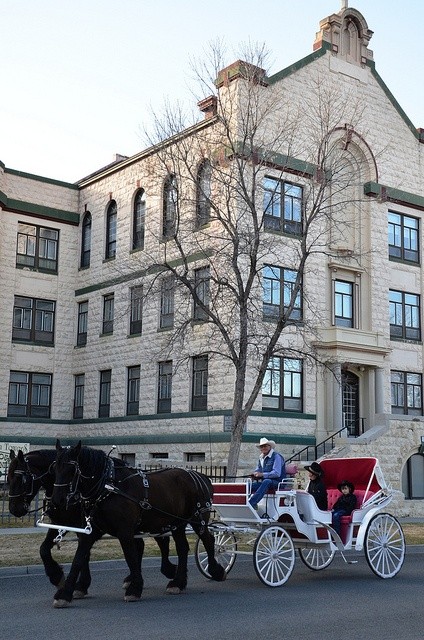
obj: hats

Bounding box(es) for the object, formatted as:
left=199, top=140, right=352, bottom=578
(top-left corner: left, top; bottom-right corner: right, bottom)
left=256, top=437, right=275, bottom=450
left=337, top=479, right=354, bottom=494
left=304, top=462, right=322, bottom=476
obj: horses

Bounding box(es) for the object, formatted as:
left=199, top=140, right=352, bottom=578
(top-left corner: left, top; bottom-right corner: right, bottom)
left=6, top=449, right=178, bottom=600
left=47, top=437, right=228, bottom=609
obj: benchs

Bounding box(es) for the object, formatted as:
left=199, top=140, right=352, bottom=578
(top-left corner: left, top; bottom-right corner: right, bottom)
left=295, top=489, right=332, bottom=541
left=246, top=485, right=295, bottom=519
left=317, top=487, right=373, bottom=544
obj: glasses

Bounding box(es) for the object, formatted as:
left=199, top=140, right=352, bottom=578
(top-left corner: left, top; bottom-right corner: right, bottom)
left=260, top=445, right=268, bottom=448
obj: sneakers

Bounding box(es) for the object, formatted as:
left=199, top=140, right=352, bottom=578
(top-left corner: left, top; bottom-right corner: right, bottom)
left=253, top=505, right=258, bottom=510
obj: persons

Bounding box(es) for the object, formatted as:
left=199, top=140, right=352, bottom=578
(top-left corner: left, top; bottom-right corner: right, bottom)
left=304, top=462, right=328, bottom=511
left=330, top=480, right=356, bottom=543
left=249, top=437, right=287, bottom=507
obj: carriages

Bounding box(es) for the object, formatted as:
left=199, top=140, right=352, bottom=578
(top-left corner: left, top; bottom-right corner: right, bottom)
left=6, top=437, right=406, bottom=608
